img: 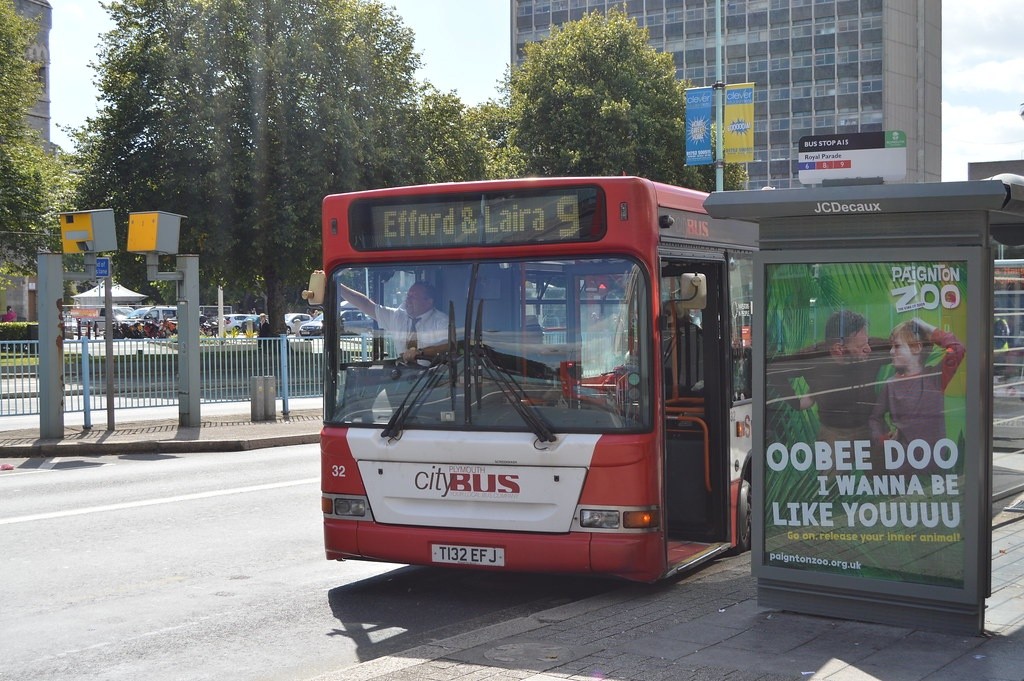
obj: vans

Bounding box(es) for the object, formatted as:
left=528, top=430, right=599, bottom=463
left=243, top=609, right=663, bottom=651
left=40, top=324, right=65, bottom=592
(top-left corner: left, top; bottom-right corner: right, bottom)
left=126, top=306, right=177, bottom=328
left=99, top=306, right=133, bottom=331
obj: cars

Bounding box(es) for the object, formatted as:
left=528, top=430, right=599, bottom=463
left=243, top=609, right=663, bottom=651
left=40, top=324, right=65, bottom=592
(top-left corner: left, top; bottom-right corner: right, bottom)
left=285, top=313, right=313, bottom=334
left=299, top=310, right=372, bottom=341
left=242, top=315, right=268, bottom=334
left=224, top=314, right=252, bottom=333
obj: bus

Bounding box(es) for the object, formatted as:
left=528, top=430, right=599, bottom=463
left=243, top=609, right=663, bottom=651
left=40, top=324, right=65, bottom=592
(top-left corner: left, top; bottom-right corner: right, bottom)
left=306, top=177, right=753, bottom=586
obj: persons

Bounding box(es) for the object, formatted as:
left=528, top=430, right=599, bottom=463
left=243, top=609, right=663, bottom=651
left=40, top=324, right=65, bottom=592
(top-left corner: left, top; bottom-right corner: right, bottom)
left=524, top=314, right=551, bottom=377
left=767, top=312, right=895, bottom=483
left=2, top=305, right=17, bottom=322
left=993, top=310, right=1010, bottom=374
left=311, top=270, right=457, bottom=369
left=307, top=309, right=319, bottom=320
left=866, top=318, right=965, bottom=481
left=256, top=312, right=272, bottom=348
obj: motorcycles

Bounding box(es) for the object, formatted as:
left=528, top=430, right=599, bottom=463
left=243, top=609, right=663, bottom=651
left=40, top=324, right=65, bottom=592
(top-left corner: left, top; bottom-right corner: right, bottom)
left=101, top=315, right=220, bottom=342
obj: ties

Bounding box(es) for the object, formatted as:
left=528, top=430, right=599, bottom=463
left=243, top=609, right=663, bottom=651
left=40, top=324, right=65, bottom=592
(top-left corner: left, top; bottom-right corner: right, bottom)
left=407, top=317, right=421, bottom=364
